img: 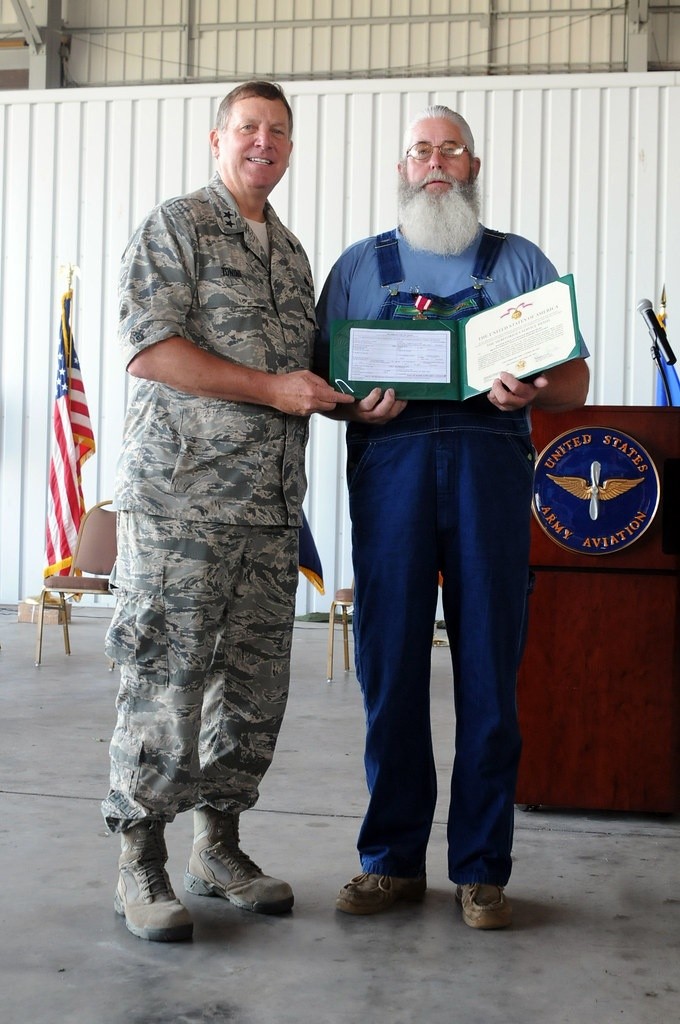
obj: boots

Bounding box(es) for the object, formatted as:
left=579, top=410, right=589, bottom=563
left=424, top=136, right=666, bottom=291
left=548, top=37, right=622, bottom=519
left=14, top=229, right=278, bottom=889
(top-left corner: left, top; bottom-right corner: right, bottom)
left=113, top=821, right=194, bottom=942
left=185, top=806, right=294, bottom=913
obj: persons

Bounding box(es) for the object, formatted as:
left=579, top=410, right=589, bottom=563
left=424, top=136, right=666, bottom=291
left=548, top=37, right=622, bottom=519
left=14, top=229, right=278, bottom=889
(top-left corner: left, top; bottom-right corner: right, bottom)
left=314, top=105, right=590, bottom=929
left=102, top=80, right=355, bottom=939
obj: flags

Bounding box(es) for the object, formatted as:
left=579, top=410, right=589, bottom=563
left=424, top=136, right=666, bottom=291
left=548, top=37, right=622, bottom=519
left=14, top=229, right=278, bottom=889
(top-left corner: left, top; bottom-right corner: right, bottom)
left=44, top=289, right=95, bottom=603
left=655, top=313, right=680, bottom=406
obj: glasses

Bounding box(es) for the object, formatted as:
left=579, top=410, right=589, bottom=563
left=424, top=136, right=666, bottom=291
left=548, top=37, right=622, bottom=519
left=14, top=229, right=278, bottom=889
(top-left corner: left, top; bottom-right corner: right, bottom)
left=405, top=141, right=470, bottom=160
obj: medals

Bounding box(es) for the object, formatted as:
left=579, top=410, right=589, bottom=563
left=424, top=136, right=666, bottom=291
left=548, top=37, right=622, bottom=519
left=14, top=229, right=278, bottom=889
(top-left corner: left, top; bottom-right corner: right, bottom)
left=411, top=312, right=427, bottom=320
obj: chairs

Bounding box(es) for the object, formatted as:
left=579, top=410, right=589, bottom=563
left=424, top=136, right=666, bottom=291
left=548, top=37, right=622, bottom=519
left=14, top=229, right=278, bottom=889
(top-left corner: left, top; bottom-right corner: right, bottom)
left=327, top=576, right=355, bottom=683
left=33, top=499, right=118, bottom=673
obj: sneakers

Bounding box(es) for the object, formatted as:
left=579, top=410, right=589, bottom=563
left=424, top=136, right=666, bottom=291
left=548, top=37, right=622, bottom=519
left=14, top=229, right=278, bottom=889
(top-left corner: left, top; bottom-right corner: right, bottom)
left=454, top=880, right=512, bottom=929
left=335, top=874, right=426, bottom=914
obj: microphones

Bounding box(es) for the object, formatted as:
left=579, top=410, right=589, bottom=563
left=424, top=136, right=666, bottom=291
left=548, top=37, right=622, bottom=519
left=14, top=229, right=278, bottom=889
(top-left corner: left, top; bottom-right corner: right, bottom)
left=636, top=298, right=677, bottom=365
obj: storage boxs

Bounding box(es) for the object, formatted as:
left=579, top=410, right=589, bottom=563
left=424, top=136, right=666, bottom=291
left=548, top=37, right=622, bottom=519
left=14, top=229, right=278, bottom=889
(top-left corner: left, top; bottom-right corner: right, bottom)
left=17, top=602, right=73, bottom=625
